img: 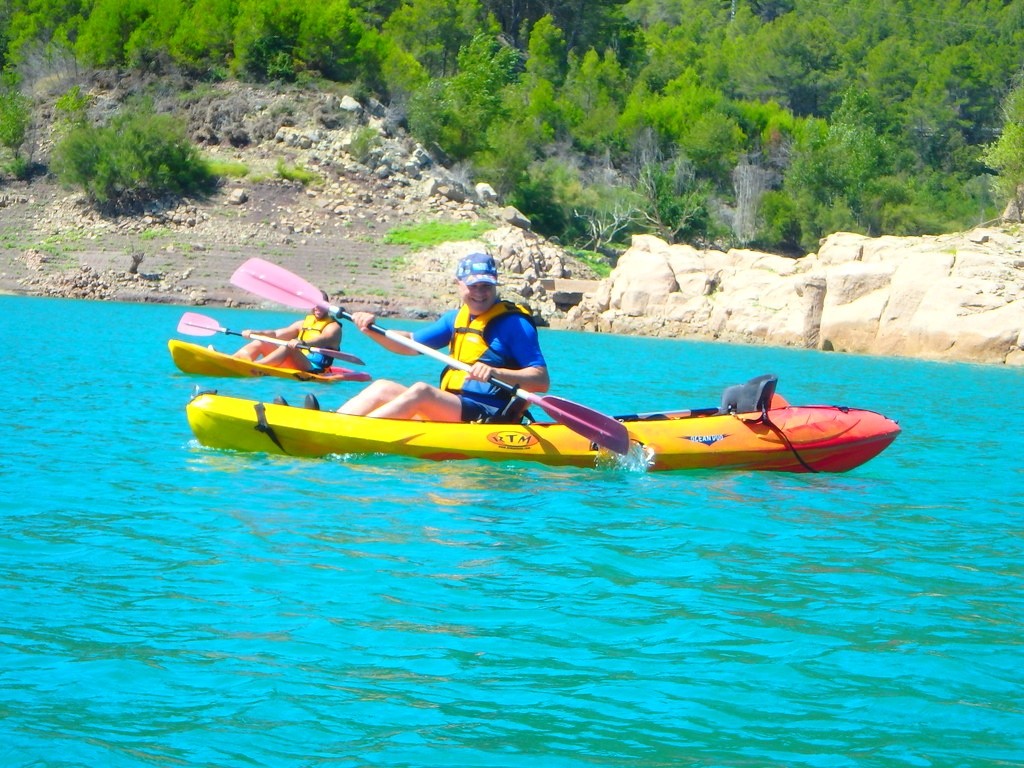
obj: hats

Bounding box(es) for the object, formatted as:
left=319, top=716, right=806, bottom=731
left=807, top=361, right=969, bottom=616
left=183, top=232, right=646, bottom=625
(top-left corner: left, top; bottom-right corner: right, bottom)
left=456, top=252, right=501, bottom=287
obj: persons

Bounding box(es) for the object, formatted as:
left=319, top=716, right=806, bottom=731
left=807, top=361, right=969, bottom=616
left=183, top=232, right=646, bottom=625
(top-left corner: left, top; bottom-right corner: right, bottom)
left=273, top=253, right=550, bottom=429
left=208, top=290, right=343, bottom=374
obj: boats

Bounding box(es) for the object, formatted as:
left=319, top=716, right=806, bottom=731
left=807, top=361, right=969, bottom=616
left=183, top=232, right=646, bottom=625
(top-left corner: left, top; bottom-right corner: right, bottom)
left=182, top=374, right=899, bottom=473
left=168, top=338, right=372, bottom=382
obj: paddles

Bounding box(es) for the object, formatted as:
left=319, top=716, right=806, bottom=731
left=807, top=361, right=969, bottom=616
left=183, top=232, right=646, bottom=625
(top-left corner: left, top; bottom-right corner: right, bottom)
left=176, top=310, right=367, bottom=367
left=226, top=255, right=632, bottom=460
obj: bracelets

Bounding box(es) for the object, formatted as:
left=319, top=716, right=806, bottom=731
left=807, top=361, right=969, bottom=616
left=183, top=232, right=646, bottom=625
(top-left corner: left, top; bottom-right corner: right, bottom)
left=301, top=340, right=306, bottom=346
